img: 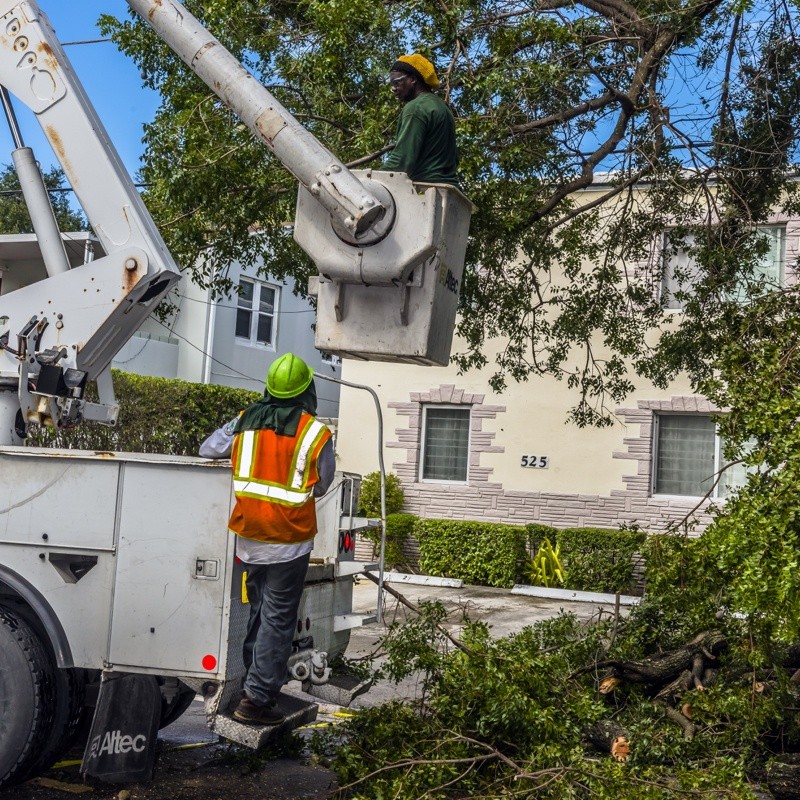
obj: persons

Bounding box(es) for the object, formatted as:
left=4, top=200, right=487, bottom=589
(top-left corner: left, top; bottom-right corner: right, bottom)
left=377, top=54, right=461, bottom=189
left=199, top=352, right=337, bottom=725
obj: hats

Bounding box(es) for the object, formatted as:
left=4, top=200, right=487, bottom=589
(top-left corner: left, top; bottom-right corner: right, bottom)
left=398, top=53, right=441, bottom=87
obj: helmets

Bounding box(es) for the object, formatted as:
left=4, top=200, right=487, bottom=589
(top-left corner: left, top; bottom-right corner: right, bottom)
left=265, top=352, right=314, bottom=399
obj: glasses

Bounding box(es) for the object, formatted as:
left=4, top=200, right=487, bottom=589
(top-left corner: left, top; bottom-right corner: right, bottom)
left=391, top=75, right=408, bottom=86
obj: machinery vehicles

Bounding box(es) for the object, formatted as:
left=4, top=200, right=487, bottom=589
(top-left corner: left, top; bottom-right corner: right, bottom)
left=0, top=1, right=473, bottom=793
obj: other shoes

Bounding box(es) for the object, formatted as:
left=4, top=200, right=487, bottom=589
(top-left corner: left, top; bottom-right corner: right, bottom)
left=233, top=694, right=285, bottom=725
